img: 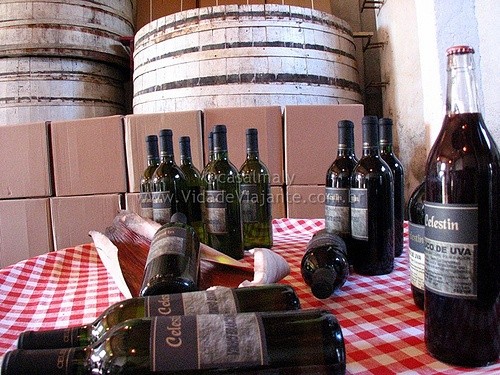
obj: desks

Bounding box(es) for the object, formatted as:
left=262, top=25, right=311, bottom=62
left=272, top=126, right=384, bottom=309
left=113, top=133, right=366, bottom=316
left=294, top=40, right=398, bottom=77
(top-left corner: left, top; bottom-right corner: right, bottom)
left=1, top=219, right=500, bottom=375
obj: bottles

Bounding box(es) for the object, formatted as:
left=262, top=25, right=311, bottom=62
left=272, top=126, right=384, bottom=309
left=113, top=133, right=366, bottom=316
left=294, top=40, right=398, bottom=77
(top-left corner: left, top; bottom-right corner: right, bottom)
left=141, top=135, right=161, bottom=221
left=139, top=212, right=201, bottom=296
left=379, top=118, right=403, bottom=257
left=324, top=120, right=360, bottom=265
left=178, top=136, right=205, bottom=244
left=425, top=45, right=500, bottom=368
left=1, top=308, right=346, bottom=375
left=300, top=227, right=349, bottom=299
left=17, top=285, right=302, bottom=349
left=408, top=182, right=426, bottom=311
left=351, top=116, right=395, bottom=276
left=152, top=128, right=188, bottom=227
left=239, top=129, right=273, bottom=250
left=201, top=131, right=214, bottom=245
left=204, top=125, right=244, bottom=260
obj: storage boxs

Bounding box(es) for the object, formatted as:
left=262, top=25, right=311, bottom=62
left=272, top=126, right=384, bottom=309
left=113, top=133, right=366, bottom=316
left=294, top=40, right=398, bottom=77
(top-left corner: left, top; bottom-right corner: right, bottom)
left=0, top=103, right=367, bottom=271
